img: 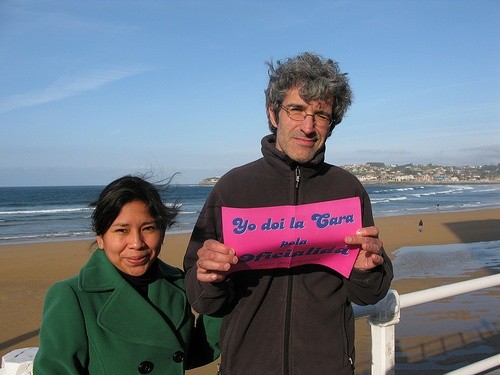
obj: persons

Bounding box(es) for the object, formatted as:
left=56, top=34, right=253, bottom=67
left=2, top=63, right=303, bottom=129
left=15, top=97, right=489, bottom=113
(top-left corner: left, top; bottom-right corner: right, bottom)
left=183, top=49, right=395, bottom=375
left=419, top=219, right=423, bottom=233
left=32, top=176, right=223, bottom=375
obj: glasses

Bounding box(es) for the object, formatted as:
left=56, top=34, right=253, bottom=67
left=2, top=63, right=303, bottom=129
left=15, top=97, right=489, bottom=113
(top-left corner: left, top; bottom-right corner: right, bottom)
left=279, top=103, right=334, bottom=128
